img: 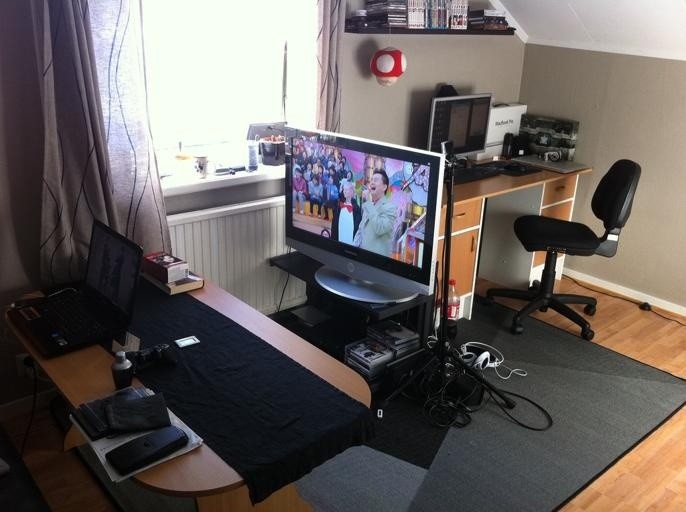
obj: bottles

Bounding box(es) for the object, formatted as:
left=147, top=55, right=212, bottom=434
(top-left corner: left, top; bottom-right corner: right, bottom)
left=442, top=279, right=460, bottom=339
left=502, top=133, right=514, bottom=156
left=246, top=146, right=258, bottom=172
left=110, top=349, right=134, bottom=389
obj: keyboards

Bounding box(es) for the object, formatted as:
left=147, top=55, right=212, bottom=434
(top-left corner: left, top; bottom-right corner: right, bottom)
left=454, top=167, right=501, bottom=185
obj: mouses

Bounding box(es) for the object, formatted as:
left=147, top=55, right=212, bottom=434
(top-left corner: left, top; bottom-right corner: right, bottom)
left=504, top=162, right=527, bottom=173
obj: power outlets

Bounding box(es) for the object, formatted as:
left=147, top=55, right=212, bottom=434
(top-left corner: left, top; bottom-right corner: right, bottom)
left=14, top=352, right=37, bottom=378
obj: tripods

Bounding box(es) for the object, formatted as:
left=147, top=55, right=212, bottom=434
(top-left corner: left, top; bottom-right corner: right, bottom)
left=380, top=139, right=517, bottom=410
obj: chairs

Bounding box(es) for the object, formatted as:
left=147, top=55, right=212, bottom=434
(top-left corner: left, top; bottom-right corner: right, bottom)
left=485, top=160, right=641, bottom=341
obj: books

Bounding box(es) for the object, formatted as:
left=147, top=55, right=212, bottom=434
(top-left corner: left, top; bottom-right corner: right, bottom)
left=364, top=0, right=508, bottom=30
left=342, top=318, right=427, bottom=390
left=77, top=384, right=145, bottom=433
left=142, top=250, right=190, bottom=285
left=139, top=269, right=205, bottom=295
left=69, top=385, right=153, bottom=443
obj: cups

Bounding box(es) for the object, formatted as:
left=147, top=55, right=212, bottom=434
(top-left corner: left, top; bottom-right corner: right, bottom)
left=193, top=156, right=207, bottom=179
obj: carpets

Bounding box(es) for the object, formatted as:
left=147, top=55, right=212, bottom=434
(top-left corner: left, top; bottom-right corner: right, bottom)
left=264, top=293, right=686, bottom=512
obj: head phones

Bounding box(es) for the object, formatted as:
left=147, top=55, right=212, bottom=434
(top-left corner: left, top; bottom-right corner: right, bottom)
left=460, top=341, right=505, bottom=371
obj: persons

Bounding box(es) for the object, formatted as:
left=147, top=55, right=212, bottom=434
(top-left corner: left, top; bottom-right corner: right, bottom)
left=96, top=242, right=109, bottom=291
left=109, top=243, right=126, bottom=305
left=290, top=137, right=401, bottom=260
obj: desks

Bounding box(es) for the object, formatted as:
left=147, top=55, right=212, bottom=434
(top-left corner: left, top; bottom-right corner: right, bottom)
left=3, top=273, right=372, bottom=511
left=433, top=164, right=594, bottom=322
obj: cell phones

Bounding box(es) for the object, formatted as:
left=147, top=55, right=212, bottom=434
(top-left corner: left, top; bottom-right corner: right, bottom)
left=104, top=425, right=189, bottom=478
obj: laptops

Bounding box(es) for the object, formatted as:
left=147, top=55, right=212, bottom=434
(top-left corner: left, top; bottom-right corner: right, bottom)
left=7, top=219, right=144, bottom=358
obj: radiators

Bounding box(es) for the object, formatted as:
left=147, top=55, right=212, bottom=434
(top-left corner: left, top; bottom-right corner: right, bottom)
left=166, top=194, right=309, bottom=317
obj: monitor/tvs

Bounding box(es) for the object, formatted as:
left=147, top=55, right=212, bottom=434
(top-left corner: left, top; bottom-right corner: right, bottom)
left=284, top=125, right=445, bottom=304
left=427, top=93, right=493, bottom=159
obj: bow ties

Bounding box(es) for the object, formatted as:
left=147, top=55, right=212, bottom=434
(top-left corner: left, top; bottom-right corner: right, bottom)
left=339, top=201, right=352, bottom=212
